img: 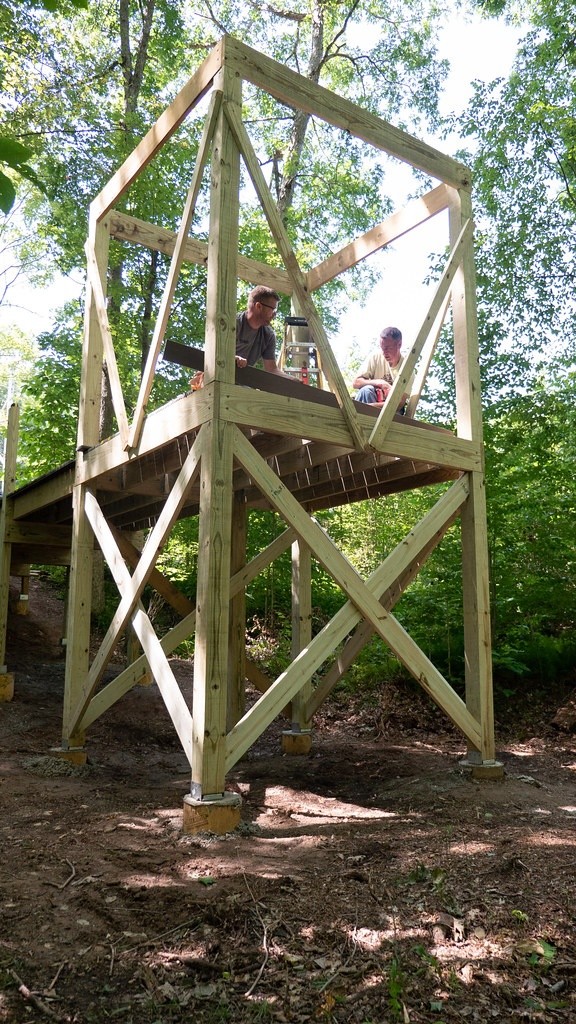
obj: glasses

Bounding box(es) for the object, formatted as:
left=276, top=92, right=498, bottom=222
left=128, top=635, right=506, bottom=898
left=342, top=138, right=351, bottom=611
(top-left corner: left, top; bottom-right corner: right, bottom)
left=261, top=303, right=277, bottom=313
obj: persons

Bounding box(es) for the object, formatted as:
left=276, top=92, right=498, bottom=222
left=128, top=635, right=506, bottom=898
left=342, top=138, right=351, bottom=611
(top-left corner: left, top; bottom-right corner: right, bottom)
left=352, top=327, right=415, bottom=416
left=234, top=285, right=306, bottom=384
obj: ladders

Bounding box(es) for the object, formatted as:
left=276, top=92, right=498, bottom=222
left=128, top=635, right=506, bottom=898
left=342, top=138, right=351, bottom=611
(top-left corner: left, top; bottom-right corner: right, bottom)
left=281, top=316, right=324, bottom=391
